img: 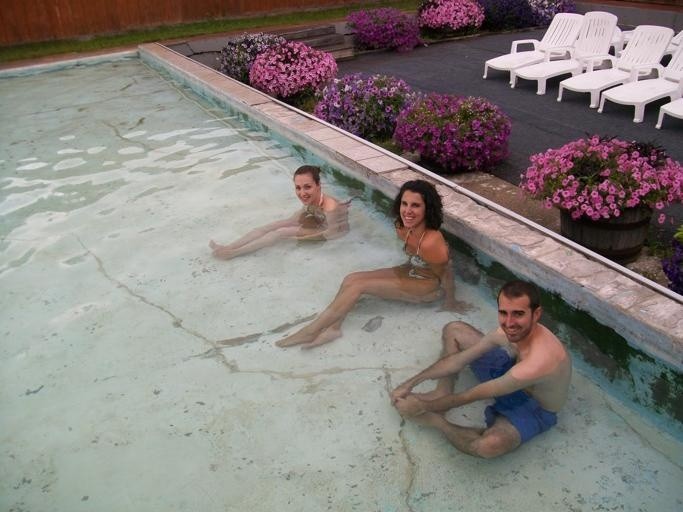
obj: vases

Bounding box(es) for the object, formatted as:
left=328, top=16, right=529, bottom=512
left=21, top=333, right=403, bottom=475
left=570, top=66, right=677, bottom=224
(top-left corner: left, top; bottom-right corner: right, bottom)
left=416, top=152, right=447, bottom=173
left=559, top=206, right=654, bottom=267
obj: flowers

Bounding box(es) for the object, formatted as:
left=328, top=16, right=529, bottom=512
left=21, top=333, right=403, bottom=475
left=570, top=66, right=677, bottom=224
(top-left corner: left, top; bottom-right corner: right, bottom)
left=390, top=92, right=512, bottom=174
left=519, top=136, right=683, bottom=226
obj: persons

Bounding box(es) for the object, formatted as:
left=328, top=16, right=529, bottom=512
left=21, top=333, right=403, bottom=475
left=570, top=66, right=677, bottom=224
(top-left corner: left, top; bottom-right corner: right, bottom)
left=208, top=165, right=350, bottom=261
left=387, top=274, right=573, bottom=460
left=272, top=179, right=474, bottom=350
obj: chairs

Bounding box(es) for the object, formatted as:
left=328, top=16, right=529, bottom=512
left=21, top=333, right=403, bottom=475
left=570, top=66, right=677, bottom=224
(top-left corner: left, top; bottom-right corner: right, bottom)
left=482, top=10, right=683, bottom=132
